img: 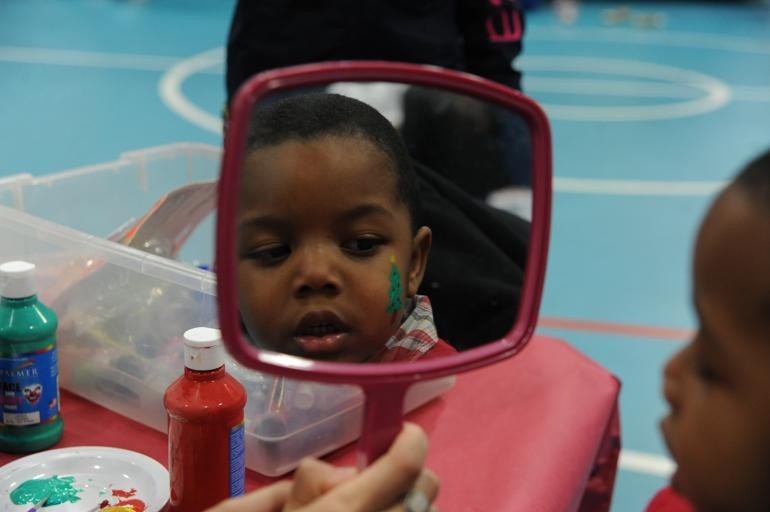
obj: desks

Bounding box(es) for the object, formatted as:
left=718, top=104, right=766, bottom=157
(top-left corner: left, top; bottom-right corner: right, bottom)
left=0, top=330, right=622, bottom=512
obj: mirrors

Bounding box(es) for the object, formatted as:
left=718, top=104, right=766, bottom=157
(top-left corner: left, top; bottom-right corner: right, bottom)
left=216, top=60, right=553, bottom=472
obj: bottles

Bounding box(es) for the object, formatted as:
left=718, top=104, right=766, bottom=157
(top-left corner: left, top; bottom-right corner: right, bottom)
left=0, top=258, right=64, bottom=454
left=165, top=324, right=247, bottom=512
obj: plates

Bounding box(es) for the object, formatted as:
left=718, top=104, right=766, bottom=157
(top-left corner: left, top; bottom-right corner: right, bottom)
left=0, top=444, right=174, bottom=512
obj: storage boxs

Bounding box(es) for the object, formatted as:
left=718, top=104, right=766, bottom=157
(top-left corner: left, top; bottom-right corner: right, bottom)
left=0, top=143, right=463, bottom=480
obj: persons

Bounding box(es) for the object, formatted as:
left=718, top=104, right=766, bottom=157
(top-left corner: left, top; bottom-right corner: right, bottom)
left=201, top=422, right=443, bottom=512
left=236, top=92, right=459, bottom=364
left=642, top=147, right=770, bottom=506
left=223, top=0, right=525, bottom=134
